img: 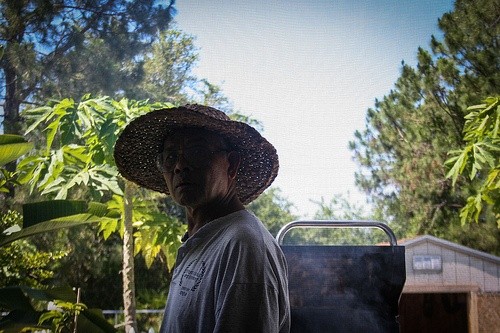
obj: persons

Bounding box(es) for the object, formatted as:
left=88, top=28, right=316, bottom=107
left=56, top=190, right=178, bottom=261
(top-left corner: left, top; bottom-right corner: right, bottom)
left=113, top=106, right=292, bottom=333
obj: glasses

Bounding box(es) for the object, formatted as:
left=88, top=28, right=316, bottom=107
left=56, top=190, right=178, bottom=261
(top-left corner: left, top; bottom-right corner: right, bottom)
left=156, top=145, right=229, bottom=173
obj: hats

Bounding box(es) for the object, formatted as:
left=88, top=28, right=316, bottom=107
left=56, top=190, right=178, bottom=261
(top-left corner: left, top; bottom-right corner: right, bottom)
left=113, top=103, right=279, bottom=206
